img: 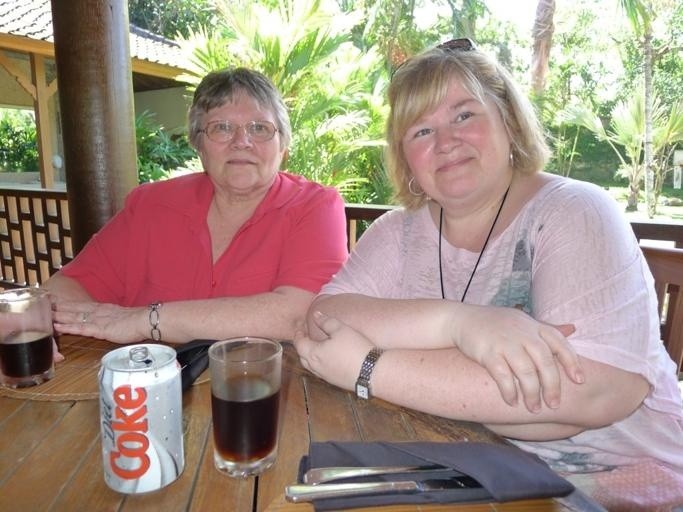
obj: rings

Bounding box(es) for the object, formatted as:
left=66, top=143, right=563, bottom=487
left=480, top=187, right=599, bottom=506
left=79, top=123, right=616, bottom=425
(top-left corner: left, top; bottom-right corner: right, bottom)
left=82, top=311, right=89, bottom=323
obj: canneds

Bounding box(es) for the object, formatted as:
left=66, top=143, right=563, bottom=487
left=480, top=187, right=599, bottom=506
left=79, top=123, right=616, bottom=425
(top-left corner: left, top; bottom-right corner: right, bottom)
left=96, top=342, right=188, bottom=495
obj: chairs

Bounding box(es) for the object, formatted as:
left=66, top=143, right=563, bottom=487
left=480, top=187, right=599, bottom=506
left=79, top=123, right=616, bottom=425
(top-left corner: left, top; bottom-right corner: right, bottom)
left=639, top=244, right=683, bottom=376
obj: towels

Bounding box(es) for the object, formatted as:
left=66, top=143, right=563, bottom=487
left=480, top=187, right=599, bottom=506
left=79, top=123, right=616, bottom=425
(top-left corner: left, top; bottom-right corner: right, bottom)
left=297, top=441, right=574, bottom=512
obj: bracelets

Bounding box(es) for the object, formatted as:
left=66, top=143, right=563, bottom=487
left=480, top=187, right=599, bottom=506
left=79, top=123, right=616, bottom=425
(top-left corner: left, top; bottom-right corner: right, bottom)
left=148, top=300, right=163, bottom=344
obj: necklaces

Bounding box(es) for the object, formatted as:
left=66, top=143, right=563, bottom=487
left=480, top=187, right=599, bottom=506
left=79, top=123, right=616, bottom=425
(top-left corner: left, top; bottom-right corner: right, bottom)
left=437, top=184, right=511, bottom=302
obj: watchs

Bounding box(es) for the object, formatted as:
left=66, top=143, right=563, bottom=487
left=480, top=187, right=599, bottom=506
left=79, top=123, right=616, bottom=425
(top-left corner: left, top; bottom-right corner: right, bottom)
left=353, top=345, right=385, bottom=397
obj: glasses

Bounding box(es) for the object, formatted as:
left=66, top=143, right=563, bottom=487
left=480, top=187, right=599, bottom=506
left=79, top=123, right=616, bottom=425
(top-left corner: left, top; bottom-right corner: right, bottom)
left=197, top=119, right=283, bottom=144
left=391, top=38, right=476, bottom=84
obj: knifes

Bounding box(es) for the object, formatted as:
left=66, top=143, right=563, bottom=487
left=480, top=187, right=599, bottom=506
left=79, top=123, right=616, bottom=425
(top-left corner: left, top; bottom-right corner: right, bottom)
left=282, top=461, right=483, bottom=504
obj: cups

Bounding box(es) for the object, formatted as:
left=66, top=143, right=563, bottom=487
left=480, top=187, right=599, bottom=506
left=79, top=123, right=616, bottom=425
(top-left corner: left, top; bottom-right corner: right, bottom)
left=0, top=288, right=53, bottom=390
left=206, top=335, right=282, bottom=478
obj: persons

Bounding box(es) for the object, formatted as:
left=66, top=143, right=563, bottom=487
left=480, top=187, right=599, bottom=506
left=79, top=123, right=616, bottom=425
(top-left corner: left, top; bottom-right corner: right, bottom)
left=289, top=36, right=681, bottom=511
left=0, top=66, right=350, bottom=361
